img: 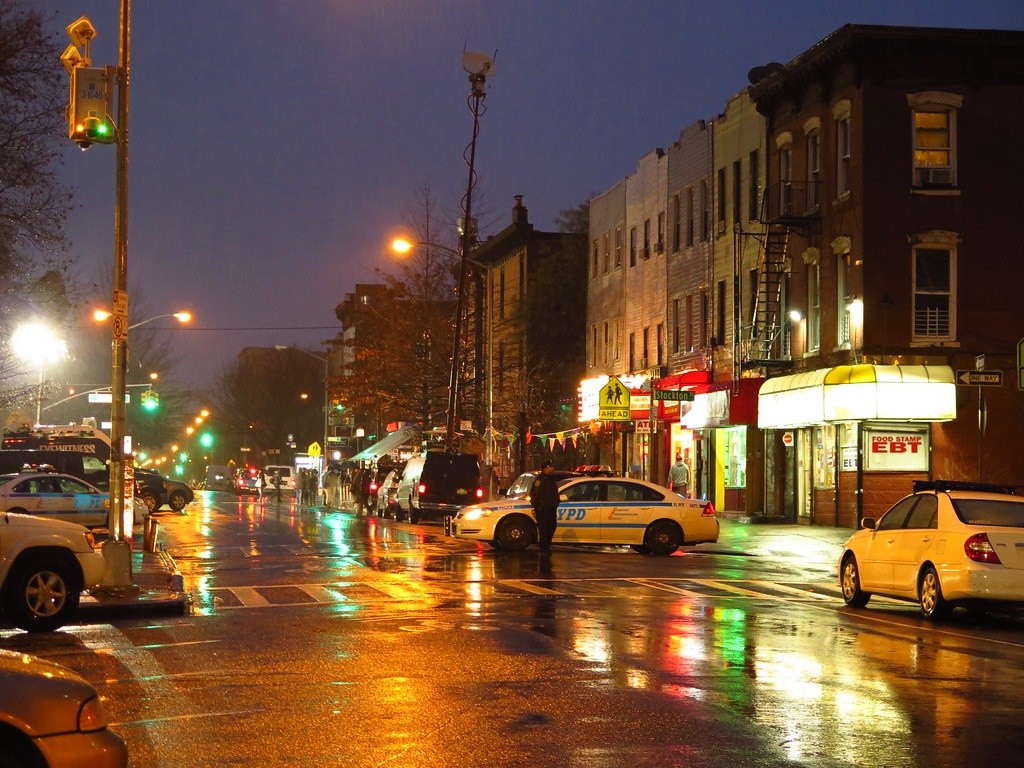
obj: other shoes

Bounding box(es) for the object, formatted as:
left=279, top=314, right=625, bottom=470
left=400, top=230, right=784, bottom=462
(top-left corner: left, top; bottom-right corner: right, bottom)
left=257, top=499, right=261, bottom=502
left=540, top=546, right=551, bottom=553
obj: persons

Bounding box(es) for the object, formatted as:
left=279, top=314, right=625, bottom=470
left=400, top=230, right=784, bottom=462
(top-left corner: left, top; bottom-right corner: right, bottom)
left=530, top=461, right=559, bottom=572
left=321, top=460, right=350, bottom=508
left=573, top=487, right=585, bottom=500
left=297, top=467, right=318, bottom=506
left=667, top=455, right=689, bottom=495
left=348, top=467, right=373, bottom=515
left=269, top=469, right=284, bottom=502
left=493, top=470, right=500, bottom=485
left=255, top=470, right=267, bottom=501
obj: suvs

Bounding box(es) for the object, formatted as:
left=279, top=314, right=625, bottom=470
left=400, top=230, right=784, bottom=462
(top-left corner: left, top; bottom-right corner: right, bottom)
left=262, top=465, right=298, bottom=497
left=370, top=452, right=483, bottom=525
left=229, top=468, right=263, bottom=495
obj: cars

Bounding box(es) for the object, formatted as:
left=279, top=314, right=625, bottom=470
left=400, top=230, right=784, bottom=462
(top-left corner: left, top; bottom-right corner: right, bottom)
left=838, top=478, right=1024, bottom=617
left=0, top=464, right=148, bottom=526
left=0, top=512, right=107, bottom=633
left=442, top=465, right=720, bottom=556
left=134, top=467, right=194, bottom=511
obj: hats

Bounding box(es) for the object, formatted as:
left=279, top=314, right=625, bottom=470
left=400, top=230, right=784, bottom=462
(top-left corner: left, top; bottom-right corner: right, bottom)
left=676, top=453, right=682, bottom=460
left=540, top=459, right=552, bottom=469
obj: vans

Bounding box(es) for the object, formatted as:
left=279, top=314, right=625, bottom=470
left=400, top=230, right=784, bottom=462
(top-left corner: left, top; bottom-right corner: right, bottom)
left=0, top=424, right=168, bottom=514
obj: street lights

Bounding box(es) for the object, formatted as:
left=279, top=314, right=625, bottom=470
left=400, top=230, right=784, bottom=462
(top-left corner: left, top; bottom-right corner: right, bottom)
left=275, top=343, right=331, bottom=469
left=11, top=320, right=59, bottom=427
left=92, top=309, right=190, bottom=540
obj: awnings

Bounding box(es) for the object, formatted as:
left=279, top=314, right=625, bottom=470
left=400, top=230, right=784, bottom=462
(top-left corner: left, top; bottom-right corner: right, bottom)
left=349, top=428, right=415, bottom=461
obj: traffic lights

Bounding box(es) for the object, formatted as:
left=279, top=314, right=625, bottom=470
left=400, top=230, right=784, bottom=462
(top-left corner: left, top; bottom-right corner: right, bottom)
left=200, top=434, right=213, bottom=448
left=141, top=392, right=159, bottom=411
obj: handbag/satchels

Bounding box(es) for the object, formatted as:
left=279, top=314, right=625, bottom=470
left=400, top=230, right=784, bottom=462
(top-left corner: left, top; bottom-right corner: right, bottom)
left=255, top=479, right=262, bottom=487
left=269, top=478, right=274, bottom=484
